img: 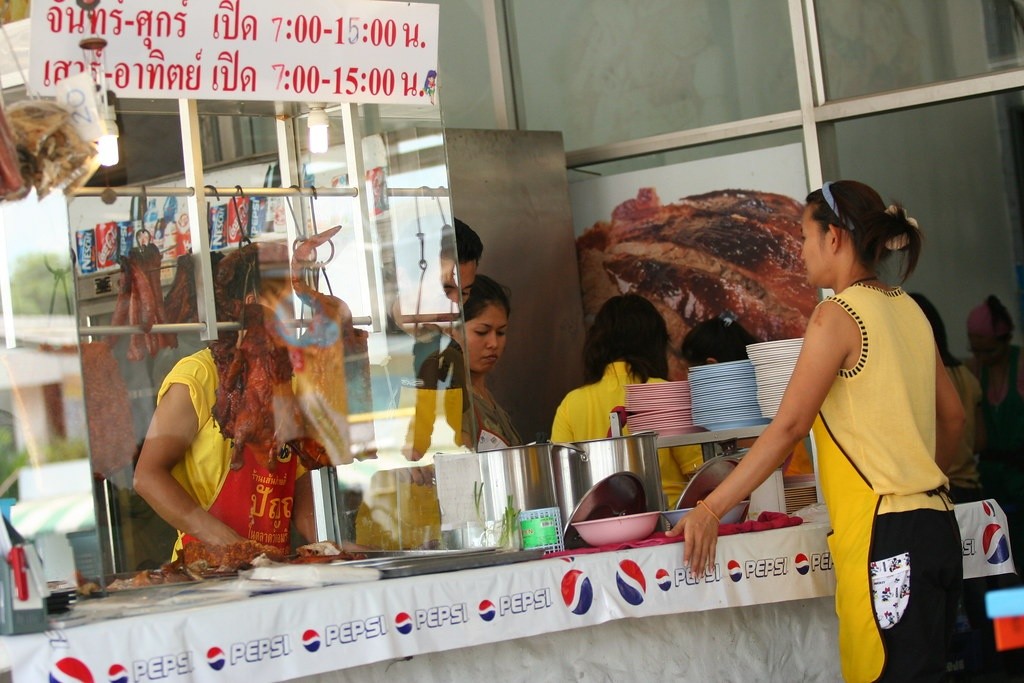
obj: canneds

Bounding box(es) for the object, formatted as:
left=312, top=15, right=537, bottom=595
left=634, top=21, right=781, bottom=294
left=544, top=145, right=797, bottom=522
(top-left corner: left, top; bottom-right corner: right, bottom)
left=75, top=166, right=390, bottom=275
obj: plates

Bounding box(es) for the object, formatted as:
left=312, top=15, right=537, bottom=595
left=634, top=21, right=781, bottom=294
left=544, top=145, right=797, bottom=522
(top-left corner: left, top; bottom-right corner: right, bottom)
left=783, top=474, right=818, bottom=517
left=621, top=338, right=805, bottom=436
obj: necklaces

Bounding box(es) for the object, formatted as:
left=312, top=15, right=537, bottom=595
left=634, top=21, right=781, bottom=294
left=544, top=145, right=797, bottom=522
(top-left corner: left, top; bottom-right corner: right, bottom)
left=850, top=276, right=877, bottom=287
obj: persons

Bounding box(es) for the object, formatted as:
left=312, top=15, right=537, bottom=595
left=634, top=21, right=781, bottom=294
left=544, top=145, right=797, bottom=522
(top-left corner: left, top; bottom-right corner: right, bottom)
left=133, top=242, right=376, bottom=564
left=550, top=294, right=703, bottom=510
left=909, top=291, right=1024, bottom=584
left=666, top=179, right=968, bottom=683
left=681, top=317, right=812, bottom=477
left=337, top=216, right=527, bottom=552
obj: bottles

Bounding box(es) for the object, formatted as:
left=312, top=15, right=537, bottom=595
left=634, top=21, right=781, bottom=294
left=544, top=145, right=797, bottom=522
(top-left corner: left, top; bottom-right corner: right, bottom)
left=144, top=199, right=157, bottom=225
left=163, top=196, right=178, bottom=224
left=128, top=195, right=148, bottom=253
left=262, top=161, right=281, bottom=233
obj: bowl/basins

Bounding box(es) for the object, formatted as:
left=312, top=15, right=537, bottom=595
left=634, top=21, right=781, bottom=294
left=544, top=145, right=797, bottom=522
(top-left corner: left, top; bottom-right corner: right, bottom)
left=570, top=510, right=660, bottom=548
left=660, top=499, right=750, bottom=528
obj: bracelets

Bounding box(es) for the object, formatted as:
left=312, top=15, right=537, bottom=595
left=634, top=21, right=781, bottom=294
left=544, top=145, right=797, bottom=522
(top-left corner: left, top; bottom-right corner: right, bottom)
left=697, top=501, right=720, bottom=522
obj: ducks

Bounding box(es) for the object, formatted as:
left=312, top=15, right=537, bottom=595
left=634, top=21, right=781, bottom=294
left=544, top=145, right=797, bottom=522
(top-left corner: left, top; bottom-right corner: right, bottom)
left=205, top=223, right=377, bottom=470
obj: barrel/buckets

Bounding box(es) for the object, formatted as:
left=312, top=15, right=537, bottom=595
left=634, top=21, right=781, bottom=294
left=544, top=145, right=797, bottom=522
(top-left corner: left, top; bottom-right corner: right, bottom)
left=552, top=431, right=664, bottom=549
left=433, top=442, right=561, bottom=551
